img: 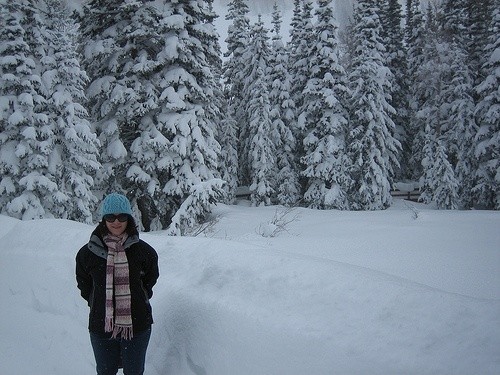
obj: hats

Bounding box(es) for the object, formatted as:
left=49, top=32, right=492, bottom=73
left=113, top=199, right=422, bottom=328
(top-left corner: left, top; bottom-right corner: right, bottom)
left=102, top=193, right=131, bottom=215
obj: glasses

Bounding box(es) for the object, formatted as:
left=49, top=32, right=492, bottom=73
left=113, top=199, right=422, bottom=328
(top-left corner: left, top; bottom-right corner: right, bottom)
left=103, top=213, right=130, bottom=223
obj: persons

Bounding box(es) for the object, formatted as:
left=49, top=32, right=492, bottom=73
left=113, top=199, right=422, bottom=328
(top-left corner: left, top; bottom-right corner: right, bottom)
left=76, top=193, right=159, bottom=375
